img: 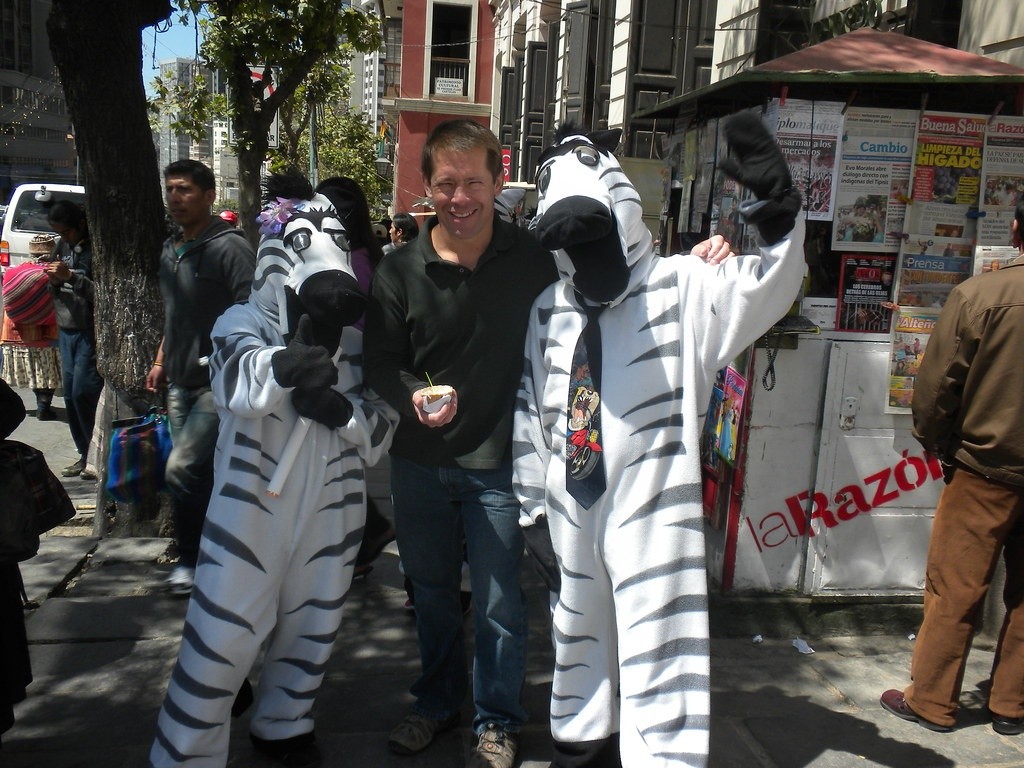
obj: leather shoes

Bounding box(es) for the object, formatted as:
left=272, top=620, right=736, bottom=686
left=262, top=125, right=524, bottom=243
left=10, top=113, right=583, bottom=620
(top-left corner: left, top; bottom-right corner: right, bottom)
left=989, top=707, right=1024, bottom=734
left=881, top=690, right=952, bottom=731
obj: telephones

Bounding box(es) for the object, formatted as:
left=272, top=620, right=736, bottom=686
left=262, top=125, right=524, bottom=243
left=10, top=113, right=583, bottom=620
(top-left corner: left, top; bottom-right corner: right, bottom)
left=771, top=314, right=817, bottom=333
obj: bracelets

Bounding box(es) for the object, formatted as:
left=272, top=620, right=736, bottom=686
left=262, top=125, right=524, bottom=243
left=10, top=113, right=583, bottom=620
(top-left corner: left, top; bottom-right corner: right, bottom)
left=154, top=362, right=163, bottom=366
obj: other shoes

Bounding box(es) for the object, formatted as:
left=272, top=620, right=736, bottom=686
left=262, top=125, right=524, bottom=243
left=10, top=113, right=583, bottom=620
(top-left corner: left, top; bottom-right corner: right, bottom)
left=405, top=599, right=415, bottom=611
left=462, top=598, right=471, bottom=615
left=81, top=470, right=96, bottom=480
left=61, top=458, right=87, bottom=476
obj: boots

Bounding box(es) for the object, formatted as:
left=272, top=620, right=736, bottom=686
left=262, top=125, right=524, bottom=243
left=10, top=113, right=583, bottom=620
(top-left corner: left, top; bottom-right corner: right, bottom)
left=34, top=388, right=57, bottom=420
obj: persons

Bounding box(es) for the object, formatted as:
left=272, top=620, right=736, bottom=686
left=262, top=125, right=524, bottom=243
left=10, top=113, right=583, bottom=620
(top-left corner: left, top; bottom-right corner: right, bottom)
left=0, top=234, right=65, bottom=422
left=878, top=198, right=1024, bottom=735
left=313, top=177, right=537, bottom=616
left=219, top=210, right=238, bottom=228
left=146, top=157, right=256, bottom=599
left=358, top=120, right=735, bottom=768
left=41, top=198, right=107, bottom=480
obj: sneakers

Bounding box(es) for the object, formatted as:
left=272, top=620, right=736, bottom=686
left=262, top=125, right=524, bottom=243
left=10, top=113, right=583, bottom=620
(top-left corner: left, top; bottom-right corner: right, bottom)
left=389, top=709, right=460, bottom=754
left=466, top=723, right=520, bottom=768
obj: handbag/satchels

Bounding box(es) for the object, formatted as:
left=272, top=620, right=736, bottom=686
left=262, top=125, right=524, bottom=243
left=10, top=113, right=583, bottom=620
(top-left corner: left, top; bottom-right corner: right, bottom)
left=107, top=384, right=170, bottom=506
left=0, top=441, right=76, bottom=560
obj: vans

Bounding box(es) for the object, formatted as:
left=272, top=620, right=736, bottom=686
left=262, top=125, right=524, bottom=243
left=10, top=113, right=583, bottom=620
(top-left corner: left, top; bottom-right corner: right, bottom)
left=0, top=183, right=87, bottom=277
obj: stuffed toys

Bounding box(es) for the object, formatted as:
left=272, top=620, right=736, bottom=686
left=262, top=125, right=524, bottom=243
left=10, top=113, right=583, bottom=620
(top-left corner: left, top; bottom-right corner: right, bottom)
left=147, top=173, right=402, bottom=768
left=505, top=104, right=809, bottom=768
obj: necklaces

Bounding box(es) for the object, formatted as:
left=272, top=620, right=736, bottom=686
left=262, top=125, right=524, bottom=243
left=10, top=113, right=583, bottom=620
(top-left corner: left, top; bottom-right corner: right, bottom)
left=179, top=233, right=197, bottom=243
